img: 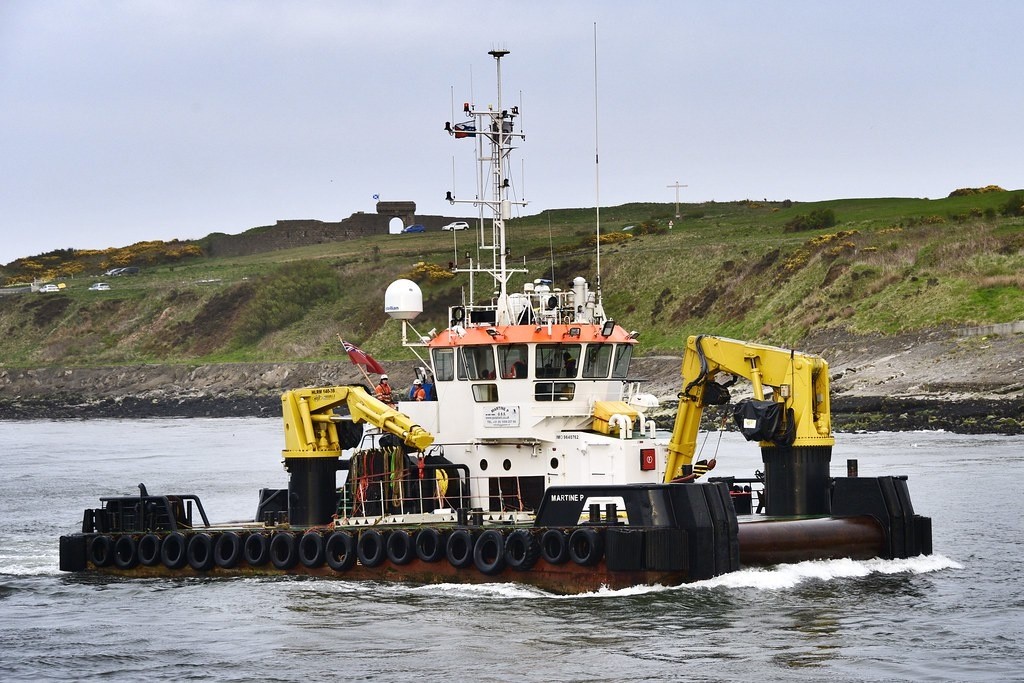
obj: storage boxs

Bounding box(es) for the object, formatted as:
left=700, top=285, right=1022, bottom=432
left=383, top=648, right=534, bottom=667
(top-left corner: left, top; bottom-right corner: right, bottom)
left=593, top=401, right=637, bottom=433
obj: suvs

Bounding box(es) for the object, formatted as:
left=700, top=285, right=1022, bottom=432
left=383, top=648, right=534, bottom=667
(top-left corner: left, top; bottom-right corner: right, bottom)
left=441, top=221, right=470, bottom=232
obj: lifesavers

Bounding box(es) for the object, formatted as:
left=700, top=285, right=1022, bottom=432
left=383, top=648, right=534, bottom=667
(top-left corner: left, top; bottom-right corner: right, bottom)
left=386, top=529, right=413, bottom=565
left=355, top=529, right=384, bottom=567
left=502, top=530, right=536, bottom=571
left=185, top=533, right=214, bottom=571
left=415, top=527, right=444, bottom=562
left=137, top=533, right=160, bottom=566
left=324, top=531, right=355, bottom=572
left=539, top=529, right=568, bottom=566
left=446, top=529, right=473, bottom=568
left=160, top=532, right=187, bottom=569
left=243, top=533, right=268, bottom=567
left=297, top=531, right=325, bottom=568
left=213, top=531, right=241, bottom=568
left=567, top=527, right=602, bottom=566
left=269, top=532, right=297, bottom=570
left=113, top=535, right=137, bottom=569
left=89, top=536, right=113, bottom=567
left=472, top=530, right=505, bottom=574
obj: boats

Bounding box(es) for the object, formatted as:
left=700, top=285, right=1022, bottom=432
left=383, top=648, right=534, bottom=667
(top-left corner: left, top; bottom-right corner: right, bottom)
left=58, top=20, right=933, bottom=594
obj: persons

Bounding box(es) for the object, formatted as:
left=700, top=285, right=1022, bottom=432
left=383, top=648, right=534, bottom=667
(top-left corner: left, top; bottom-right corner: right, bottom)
left=482, top=370, right=489, bottom=379
left=733, top=485, right=751, bottom=492
left=376, top=374, right=396, bottom=410
left=561, top=352, right=571, bottom=376
left=413, top=379, right=426, bottom=401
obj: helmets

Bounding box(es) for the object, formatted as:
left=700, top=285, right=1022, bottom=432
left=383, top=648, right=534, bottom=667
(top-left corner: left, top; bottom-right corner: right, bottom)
left=413, top=379, right=421, bottom=385
left=381, top=375, right=388, bottom=380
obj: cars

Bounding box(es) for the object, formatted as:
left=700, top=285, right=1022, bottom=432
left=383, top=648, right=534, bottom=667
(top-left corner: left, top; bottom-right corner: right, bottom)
left=39, top=284, right=60, bottom=292
left=104, top=268, right=140, bottom=276
left=402, top=224, right=426, bottom=234
left=88, top=283, right=111, bottom=290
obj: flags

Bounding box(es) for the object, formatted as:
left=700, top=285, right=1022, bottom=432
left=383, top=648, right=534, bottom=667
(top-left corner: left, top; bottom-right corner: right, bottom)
left=343, top=340, right=384, bottom=374
